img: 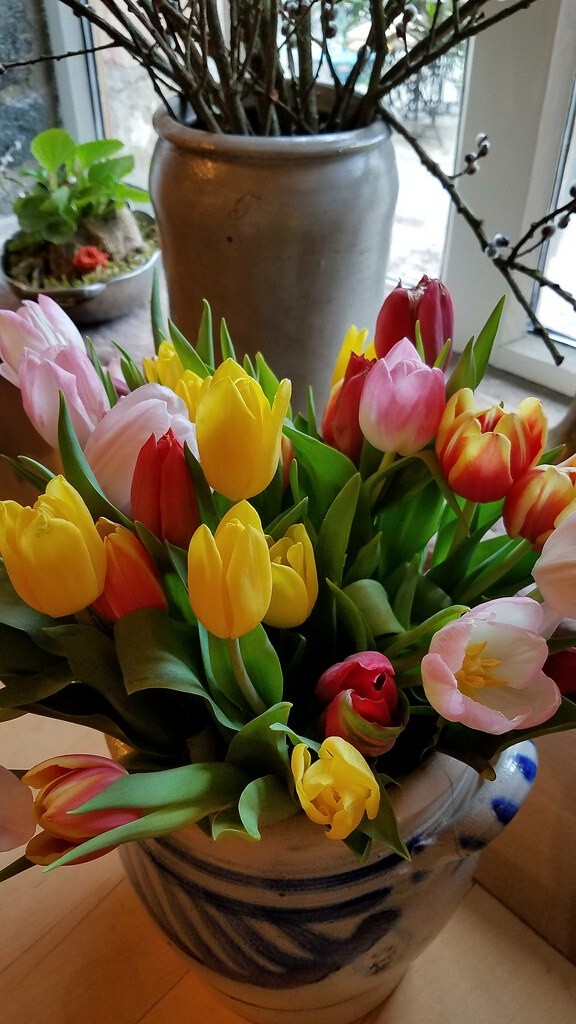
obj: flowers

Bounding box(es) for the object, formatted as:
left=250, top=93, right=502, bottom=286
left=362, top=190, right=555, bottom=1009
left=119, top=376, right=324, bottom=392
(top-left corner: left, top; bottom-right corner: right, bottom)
left=72, top=244, right=109, bottom=273
left=58, top=0, right=575, bottom=364
left=0, top=276, right=575, bottom=878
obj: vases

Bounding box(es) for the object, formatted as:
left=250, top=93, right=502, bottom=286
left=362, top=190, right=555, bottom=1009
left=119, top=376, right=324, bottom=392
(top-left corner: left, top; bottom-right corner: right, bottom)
left=146, top=76, right=401, bottom=437
left=114, top=737, right=541, bottom=1024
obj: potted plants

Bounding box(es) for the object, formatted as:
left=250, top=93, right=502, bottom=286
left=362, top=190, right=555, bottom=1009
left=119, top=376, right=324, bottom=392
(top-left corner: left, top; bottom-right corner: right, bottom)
left=0, top=126, right=164, bottom=326
